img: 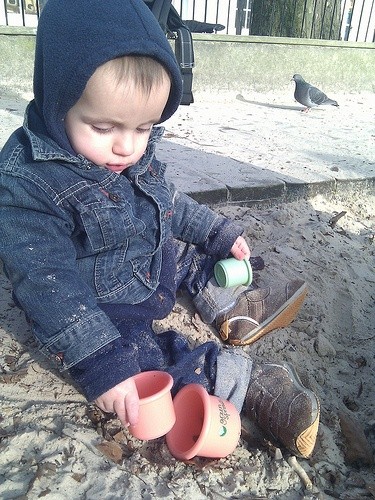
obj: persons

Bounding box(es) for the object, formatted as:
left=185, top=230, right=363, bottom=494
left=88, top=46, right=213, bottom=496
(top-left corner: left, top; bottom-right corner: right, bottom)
left=0, top=0, right=319, bottom=462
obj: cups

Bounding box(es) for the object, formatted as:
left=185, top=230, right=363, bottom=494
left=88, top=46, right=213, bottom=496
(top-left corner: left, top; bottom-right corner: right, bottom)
left=166, top=383, right=242, bottom=462
left=215, top=256, right=253, bottom=289
left=123, top=369, right=175, bottom=440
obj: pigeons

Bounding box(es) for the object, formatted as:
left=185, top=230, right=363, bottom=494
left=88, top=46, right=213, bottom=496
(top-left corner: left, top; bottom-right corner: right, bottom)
left=290, top=74, right=339, bottom=113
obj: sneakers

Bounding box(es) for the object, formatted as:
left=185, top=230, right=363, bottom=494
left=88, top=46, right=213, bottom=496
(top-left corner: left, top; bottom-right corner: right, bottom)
left=215, top=279, right=307, bottom=346
left=244, top=359, right=320, bottom=459
